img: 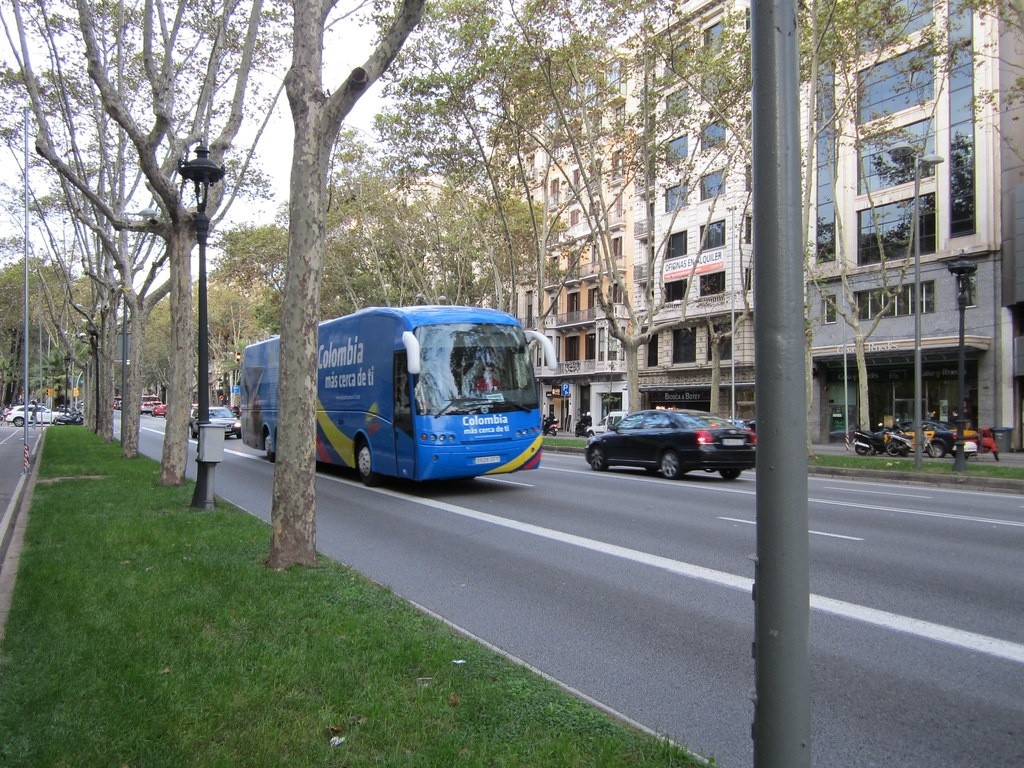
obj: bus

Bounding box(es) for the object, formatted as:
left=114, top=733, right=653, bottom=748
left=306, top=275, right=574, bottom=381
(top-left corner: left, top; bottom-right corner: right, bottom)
left=140, top=396, right=160, bottom=403
left=237, top=304, right=560, bottom=488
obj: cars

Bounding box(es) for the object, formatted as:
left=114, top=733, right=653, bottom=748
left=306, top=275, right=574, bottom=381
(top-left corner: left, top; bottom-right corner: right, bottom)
left=584, top=410, right=757, bottom=480
left=142, top=402, right=166, bottom=417
left=190, top=406, right=241, bottom=439
left=114, top=400, right=122, bottom=410
left=873, top=419, right=978, bottom=458
left=7, top=405, right=67, bottom=427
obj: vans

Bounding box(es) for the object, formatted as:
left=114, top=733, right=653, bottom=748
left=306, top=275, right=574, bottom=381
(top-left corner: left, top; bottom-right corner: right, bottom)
left=587, top=411, right=628, bottom=438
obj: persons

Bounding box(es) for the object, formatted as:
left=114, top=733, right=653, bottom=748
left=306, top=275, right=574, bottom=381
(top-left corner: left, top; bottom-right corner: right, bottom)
left=1, top=404, right=11, bottom=427
left=473, top=361, right=504, bottom=392
left=31, top=403, right=39, bottom=429
left=544, top=412, right=555, bottom=433
left=578, top=411, right=592, bottom=433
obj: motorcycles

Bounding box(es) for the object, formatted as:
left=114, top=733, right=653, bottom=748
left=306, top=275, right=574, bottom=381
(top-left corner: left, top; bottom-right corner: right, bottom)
left=54, top=409, right=83, bottom=425
left=852, top=419, right=909, bottom=456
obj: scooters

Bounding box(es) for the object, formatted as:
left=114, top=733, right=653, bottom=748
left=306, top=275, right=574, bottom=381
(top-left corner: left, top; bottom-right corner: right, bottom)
left=952, top=424, right=1000, bottom=462
left=886, top=425, right=936, bottom=459
left=575, top=419, right=592, bottom=438
left=544, top=418, right=559, bottom=436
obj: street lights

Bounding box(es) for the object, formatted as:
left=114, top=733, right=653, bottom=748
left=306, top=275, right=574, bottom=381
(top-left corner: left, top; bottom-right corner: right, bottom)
left=887, top=142, right=944, bottom=470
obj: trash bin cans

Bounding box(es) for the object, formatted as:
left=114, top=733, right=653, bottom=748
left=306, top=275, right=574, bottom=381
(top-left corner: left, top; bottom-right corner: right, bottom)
left=979, top=427, right=996, bottom=454
left=991, top=427, right=1014, bottom=453
